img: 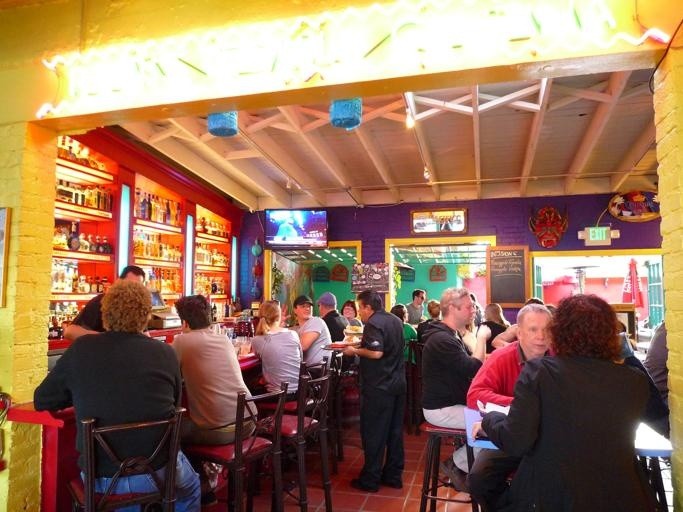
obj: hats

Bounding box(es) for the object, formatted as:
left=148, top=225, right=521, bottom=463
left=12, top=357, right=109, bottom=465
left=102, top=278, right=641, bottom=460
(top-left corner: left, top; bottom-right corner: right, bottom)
left=294, top=295, right=314, bottom=306
left=317, top=293, right=336, bottom=305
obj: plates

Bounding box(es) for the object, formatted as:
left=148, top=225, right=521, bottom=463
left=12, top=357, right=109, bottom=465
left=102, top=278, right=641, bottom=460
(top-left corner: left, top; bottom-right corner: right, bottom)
left=320, top=344, right=361, bottom=350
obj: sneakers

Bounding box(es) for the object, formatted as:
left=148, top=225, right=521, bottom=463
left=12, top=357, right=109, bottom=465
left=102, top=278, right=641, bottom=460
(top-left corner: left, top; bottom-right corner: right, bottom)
left=440, top=456, right=467, bottom=491
left=202, top=490, right=218, bottom=506
left=379, top=476, right=403, bottom=489
left=351, top=479, right=379, bottom=493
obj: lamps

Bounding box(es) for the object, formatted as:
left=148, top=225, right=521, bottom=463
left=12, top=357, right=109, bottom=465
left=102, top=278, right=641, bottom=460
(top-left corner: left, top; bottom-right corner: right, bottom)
left=423, top=165, right=431, bottom=182
left=403, top=108, right=415, bottom=127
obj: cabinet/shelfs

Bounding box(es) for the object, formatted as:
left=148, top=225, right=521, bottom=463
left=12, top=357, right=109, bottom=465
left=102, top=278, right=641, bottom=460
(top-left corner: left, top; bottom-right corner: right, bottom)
left=48, top=162, right=115, bottom=351
left=134, top=175, right=183, bottom=314
left=194, top=204, right=230, bottom=321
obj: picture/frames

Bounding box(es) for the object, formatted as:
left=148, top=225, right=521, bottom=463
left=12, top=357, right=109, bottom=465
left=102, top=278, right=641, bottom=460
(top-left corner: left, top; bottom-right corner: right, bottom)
left=410, top=209, right=469, bottom=237
left=0, top=206, right=14, bottom=308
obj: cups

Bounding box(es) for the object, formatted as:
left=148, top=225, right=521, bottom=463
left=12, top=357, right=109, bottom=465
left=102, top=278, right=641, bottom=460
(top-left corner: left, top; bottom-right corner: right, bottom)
left=230, top=335, right=253, bottom=356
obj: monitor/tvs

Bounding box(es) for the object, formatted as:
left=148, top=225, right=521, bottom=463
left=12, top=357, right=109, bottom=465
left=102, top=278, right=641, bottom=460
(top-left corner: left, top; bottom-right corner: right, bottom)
left=264, top=208, right=329, bottom=249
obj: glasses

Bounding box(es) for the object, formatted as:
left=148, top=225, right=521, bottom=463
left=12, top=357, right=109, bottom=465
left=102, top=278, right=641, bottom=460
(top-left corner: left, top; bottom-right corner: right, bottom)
left=419, top=296, right=424, bottom=299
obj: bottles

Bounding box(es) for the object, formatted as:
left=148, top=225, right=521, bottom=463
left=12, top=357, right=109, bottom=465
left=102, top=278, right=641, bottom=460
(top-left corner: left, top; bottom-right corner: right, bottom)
left=48, top=177, right=112, bottom=339
left=212, top=304, right=217, bottom=322
left=225, top=300, right=229, bottom=317
left=134, top=187, right=183, bottom=304
left=193, top=217, right=228, bottom=294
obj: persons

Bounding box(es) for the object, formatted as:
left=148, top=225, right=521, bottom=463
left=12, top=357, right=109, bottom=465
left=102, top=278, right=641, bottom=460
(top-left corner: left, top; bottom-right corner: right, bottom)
left=33, top=265, right=667, bottom=512
left=439, top=216, right=462, bottom=232
left=275, top=217, right=297, bottom=241
